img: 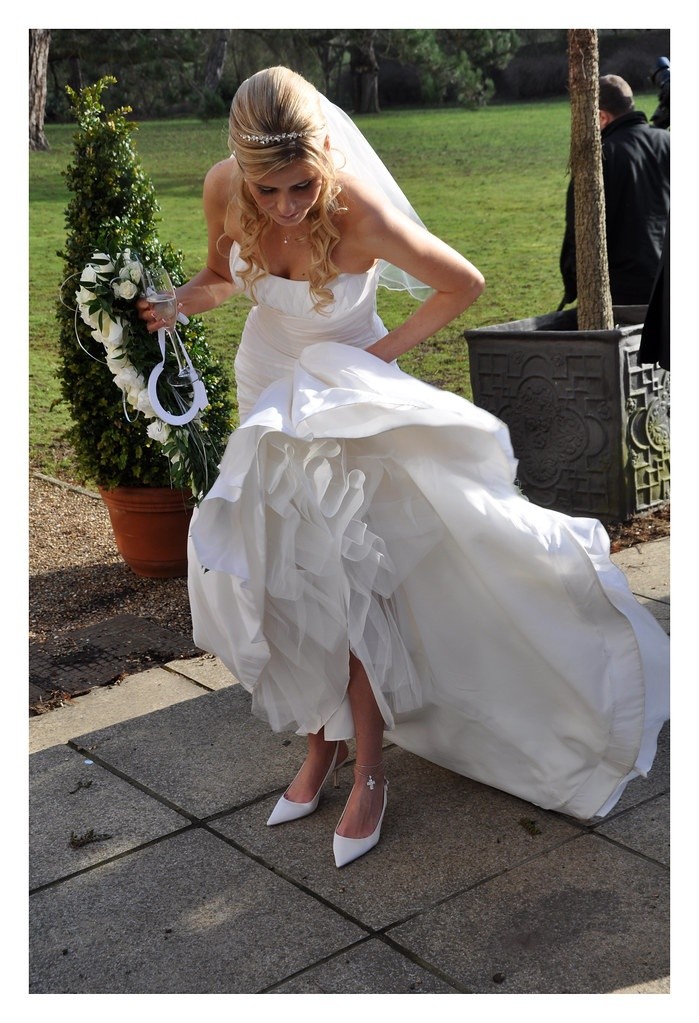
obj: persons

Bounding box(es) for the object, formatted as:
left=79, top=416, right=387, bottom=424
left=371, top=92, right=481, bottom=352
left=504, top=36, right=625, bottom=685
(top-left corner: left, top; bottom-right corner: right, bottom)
left=558, top=56, right=670, bottom=372
left=137, top=65, right=671, bottom=866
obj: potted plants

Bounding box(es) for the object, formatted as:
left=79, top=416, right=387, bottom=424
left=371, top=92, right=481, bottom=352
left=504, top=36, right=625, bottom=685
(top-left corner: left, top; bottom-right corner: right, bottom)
left=54, top=77, right=241, bottom=579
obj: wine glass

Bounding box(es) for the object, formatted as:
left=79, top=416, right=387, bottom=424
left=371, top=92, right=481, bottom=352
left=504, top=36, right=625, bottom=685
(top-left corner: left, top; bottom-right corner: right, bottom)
left=141, top=267, right=203, bottom=388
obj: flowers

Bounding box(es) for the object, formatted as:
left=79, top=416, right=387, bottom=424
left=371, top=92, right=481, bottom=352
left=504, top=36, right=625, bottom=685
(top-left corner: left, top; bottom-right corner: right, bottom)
left=59, top=245, right=226, bottom=507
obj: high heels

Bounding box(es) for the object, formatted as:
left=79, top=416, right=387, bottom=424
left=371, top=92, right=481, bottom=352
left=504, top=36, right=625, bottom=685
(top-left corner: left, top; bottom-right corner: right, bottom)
left=267, top=740, right=350, bottom=827
left=333, top=774, right=388, bottom=868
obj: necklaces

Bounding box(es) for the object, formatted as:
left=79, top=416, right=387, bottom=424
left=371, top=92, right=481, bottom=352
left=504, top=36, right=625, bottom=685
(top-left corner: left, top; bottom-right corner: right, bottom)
left=274, top=220, right=294, bottom=244
